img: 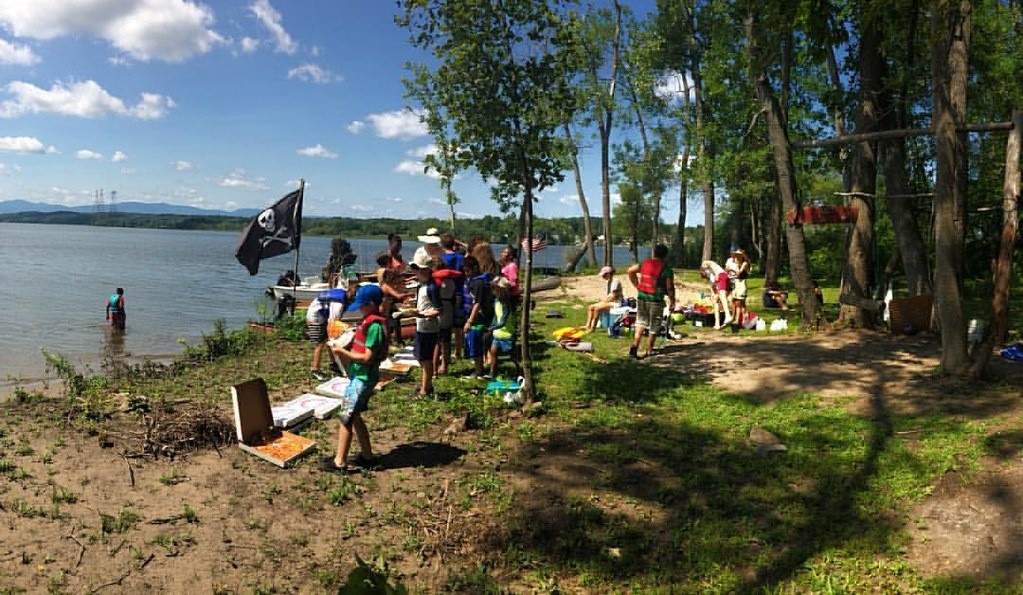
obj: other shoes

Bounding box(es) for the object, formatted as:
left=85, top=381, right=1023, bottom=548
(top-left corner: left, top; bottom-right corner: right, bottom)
left=347, top=453, right=373, bottom=467
left=318, top=454, right=348, bottom=472
left=406, top=387, right=434, bottom=402
left=311, top=370, right=329, bottom=382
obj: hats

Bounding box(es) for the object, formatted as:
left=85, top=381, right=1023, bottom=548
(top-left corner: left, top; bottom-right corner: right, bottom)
left=348, top=284, right=383, bottom=312
left=597, top=267, right=614, bottom=277
left=417, top=228, right=441, bottom=244
left=408, top=257, right=433, bottom=271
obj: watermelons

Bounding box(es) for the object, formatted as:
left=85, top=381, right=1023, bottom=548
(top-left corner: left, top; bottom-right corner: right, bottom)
left=671, top=313, right=686, bottom=324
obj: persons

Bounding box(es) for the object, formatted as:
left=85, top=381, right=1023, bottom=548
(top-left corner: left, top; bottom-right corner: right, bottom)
left=700, top=260, right=733, bottom=330
left=580, top=266, right=622, bottom=333
left=724, top=248, right=751, bottom=327
left=316, top=285, right=391, bottom=474
left=762, top=281, right=790, bottom=311
left=105, top=287, right=126, bottom=330
left=305, top=283, right=362, bottom=379
left=273, top=271, right=302, bottom=318
left=812, top=280, right=823, bottom=306
left=376, top=224, right=523, bottom=398
left=626, top=244, right=676, bottom=359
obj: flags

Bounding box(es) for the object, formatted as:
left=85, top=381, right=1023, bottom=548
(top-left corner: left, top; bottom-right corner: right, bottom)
left=520, top=231, right=548, bottom=253
left=234, top=187, right=304, bottom=277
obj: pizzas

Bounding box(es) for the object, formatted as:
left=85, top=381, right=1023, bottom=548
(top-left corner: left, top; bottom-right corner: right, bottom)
left=251, top=432, right=303, bottom=461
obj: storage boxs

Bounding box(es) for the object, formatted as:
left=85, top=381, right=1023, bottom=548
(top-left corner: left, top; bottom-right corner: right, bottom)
left=600, top=306, right=630, bottom=329
left=230, top=376, right=350, bottom=469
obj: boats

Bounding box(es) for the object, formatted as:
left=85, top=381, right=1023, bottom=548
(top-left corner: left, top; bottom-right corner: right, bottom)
left=268, top=271, right=379, bottom=304
